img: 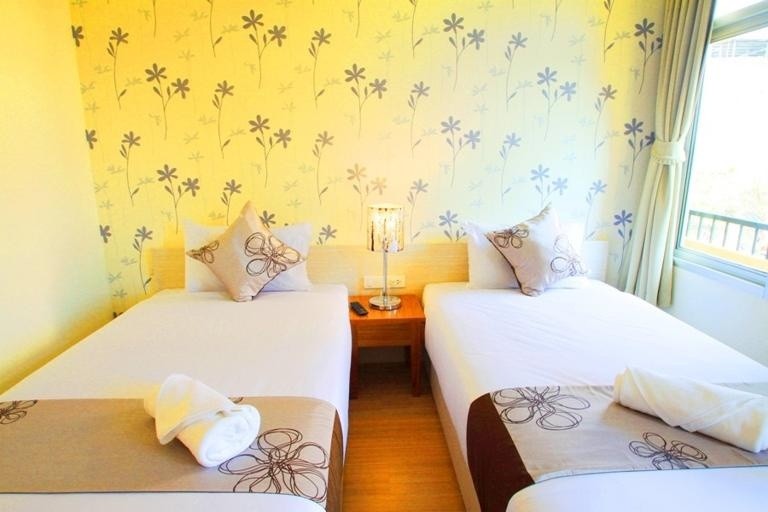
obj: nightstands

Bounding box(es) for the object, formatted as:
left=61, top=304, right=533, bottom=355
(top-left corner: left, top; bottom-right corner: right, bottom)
left=347, top=295, right=425, bottom=397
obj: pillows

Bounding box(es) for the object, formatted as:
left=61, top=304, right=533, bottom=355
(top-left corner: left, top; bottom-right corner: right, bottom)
left=466, top=219, right=585, bottom=290
left=488, top=202, right=589, bottom=296
left=186, top=219, right=313, bottom=291
left=186, top=200, right=308, bottom=301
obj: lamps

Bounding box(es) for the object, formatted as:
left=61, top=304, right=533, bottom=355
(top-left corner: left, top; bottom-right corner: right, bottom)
left=366, top=204, right=406, bottom=310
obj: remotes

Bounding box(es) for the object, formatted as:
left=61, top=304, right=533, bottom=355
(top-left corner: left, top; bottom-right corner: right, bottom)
left=350, top=301, right=368, bottom=316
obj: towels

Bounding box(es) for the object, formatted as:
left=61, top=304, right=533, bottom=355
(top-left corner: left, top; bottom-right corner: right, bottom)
left=614, top=368, right=767, bottom=453
left=144, top=373, right=261, bottom=467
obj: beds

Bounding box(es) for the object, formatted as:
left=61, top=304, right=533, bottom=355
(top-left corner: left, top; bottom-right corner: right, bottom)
left=414, top=238, right=767, bottom=512
left=0, top=244, right=353, bottom=512
